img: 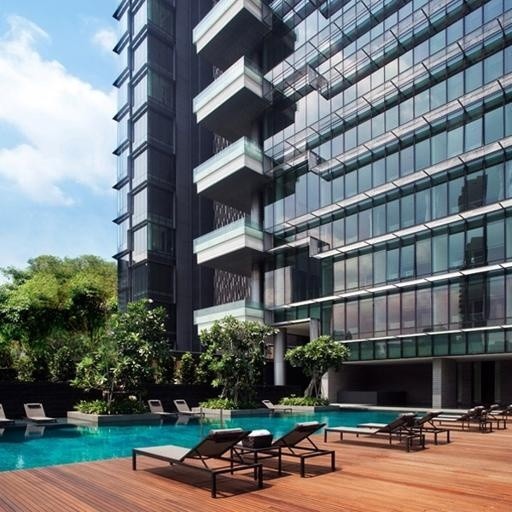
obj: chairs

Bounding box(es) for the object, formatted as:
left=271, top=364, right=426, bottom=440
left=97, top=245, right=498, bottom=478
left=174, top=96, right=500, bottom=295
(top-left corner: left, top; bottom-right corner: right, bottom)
left=324, top=404, right=512, bottom=452
left=261, top=399, right=292, bottom=413
left=0, top=402, right=57, bottom=426
left=147, top=398, right=206, bottom=418
left=131, top=421, right=336, bottom=498
